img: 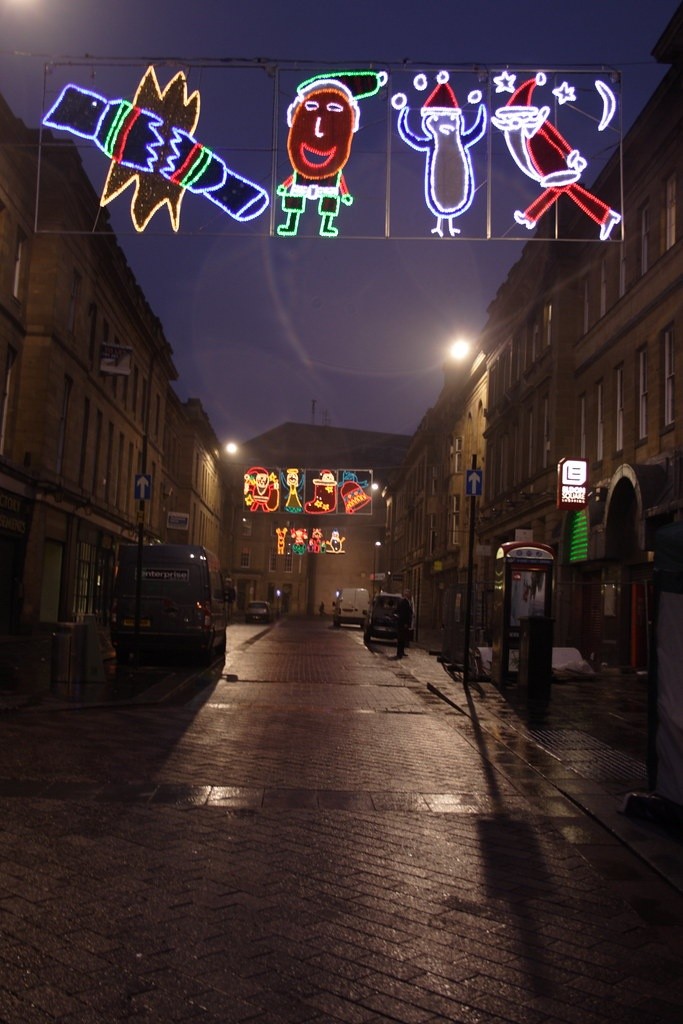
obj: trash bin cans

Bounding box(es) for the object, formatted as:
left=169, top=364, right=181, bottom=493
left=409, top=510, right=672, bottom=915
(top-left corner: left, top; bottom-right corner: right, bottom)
left=518, top=615, right=555, bottom=689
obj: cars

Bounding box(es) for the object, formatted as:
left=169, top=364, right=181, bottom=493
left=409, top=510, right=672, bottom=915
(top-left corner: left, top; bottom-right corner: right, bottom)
left=246, top=601, right=272, bottom=623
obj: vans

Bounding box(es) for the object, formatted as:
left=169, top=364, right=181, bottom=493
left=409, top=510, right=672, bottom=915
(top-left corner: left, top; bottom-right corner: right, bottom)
left=332, top=588, right=369, bottom=627
left=364, top=593, right=414, bottom=646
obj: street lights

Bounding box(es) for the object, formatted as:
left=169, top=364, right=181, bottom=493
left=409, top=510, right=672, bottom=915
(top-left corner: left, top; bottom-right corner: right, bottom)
left=373, top=540, right=382, bottom=597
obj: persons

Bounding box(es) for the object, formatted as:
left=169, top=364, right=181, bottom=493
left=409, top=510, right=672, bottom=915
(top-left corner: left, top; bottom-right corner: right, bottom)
left=319, top=601, right=325, bottom=616
left=396, top=589, right=413, bottom=659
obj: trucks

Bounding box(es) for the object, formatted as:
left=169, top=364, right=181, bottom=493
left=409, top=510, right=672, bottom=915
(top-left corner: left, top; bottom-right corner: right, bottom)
left=110, top=544, right=236, bottom=670
